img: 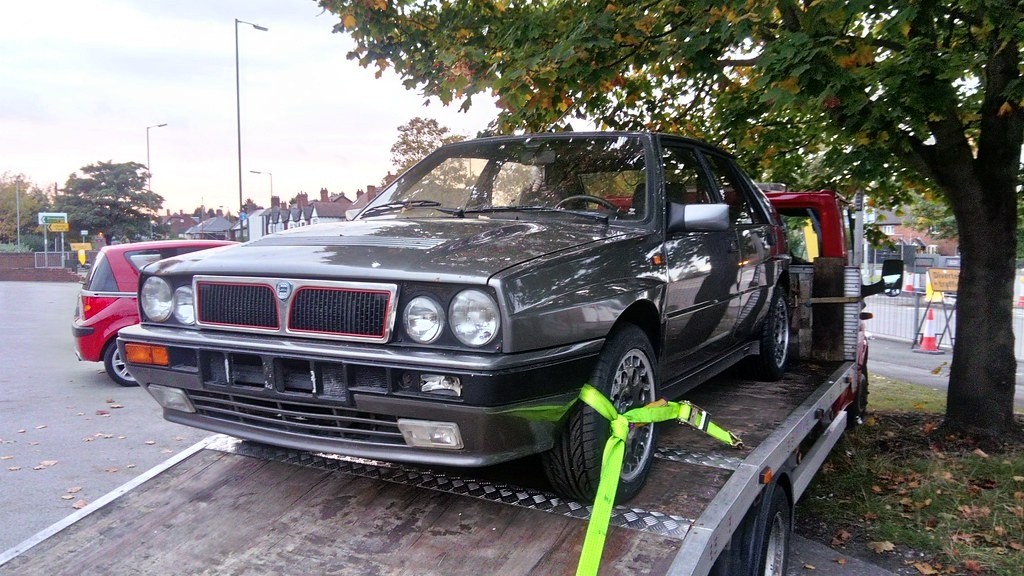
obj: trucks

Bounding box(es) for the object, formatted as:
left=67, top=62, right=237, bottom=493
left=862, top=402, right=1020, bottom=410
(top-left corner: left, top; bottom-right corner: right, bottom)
left=0, top=194, right=903, bottom=575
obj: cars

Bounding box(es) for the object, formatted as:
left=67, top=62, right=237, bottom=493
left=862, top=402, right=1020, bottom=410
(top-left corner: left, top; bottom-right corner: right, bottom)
left=71, top=236, right=242, bottom=387
left=117, top=132, right=793, bottom=505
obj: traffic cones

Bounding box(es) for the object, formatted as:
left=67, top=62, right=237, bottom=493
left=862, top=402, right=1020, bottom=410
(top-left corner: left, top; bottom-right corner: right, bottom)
left=910, top=308, right=946, bottom=355
left=1013, top=282, right=1024, bottom=309
left=903, top=272, right=916, bottom=293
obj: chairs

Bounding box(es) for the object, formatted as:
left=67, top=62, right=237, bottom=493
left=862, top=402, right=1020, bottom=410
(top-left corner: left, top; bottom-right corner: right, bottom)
left=518, top=186, right=572, bottom=211
left=624, top=183, right=687, bottom=221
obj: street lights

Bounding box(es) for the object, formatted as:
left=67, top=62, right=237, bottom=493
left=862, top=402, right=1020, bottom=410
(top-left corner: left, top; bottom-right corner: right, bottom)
left=250, top=170, right=272, bottom=197
left=235, top=19, right=270, bottom=243
left=147, top=123, right=167, bottom=241
